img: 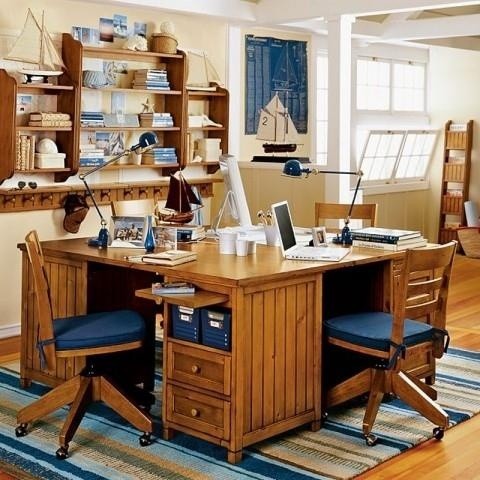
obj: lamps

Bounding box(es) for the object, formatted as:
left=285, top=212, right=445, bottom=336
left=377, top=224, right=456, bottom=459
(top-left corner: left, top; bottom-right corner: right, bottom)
left=78, top=132, right=160, bottom=248
left=282, top=160, right=364, bottom=244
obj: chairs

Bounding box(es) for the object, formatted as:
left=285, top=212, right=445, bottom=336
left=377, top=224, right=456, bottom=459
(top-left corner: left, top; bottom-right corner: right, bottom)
left=321, top=239, right=459, bottom=447
left=314, top=201, right=378, bottom=234
left=15, top=228, right=155, bottom=461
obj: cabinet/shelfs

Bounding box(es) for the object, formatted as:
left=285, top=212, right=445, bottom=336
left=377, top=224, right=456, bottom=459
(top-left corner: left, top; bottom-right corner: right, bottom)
left=1, top=32, right=230, bottom=183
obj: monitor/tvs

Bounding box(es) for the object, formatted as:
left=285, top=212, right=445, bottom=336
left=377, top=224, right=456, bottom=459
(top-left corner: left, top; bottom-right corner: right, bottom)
left=212, top=155, right=252, bottom=237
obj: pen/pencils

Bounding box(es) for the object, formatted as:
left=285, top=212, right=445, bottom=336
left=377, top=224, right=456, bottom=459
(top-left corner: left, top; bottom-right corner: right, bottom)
left=258, top=209, right=272, bottom=226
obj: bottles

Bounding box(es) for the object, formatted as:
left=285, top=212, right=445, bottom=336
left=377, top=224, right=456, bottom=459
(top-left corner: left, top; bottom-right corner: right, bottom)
left=144, top=216, right=156, bottom=252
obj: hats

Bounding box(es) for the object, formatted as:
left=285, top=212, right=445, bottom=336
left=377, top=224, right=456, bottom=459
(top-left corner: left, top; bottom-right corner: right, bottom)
left=63, top=194, right=89, bottom=233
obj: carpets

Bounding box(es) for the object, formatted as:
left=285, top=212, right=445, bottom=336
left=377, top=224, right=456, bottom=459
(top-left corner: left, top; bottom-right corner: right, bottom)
left=0, top=342, right=480, bottom=480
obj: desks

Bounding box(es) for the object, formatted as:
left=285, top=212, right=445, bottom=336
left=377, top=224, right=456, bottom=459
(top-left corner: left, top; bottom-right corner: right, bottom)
left=17, top=226, right=442, bottom=465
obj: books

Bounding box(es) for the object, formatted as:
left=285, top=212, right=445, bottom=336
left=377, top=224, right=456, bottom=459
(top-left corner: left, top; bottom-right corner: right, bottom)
left=351, top=238, right=429, bottom=251
left=348, top=235, right=425, bottom=245
left=177, top=230, right=207, bottom=241
left=150, top=281, right=195, bottom=294
left=79, top=144, right=105, bottom=166
left=27, top=111, right=70, bottom=122
left=141, top=249, right=198, bottom=267
left=28, top=120, right=73, bottom=127
left=141, top=147, right=177, bottom=165
left=80, top=110, right=108, bottom=130
left=16, top=131, right=36, bottom=171
left=131, top=69, right=171, bottom=91
left=348, top=227, right=421, bottom=241
left=178, top=226, right=206, bottom=232
left=139, top=112, right=174, bottom=128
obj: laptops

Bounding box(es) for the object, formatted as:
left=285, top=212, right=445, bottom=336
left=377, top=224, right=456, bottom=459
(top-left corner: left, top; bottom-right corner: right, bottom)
left=271, top=200, right=351, bottom=262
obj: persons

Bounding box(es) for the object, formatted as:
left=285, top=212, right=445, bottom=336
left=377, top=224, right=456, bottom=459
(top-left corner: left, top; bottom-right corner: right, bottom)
left=116, top=223, right=139, bottom=241
left=318, top=232, right=324, bottom=243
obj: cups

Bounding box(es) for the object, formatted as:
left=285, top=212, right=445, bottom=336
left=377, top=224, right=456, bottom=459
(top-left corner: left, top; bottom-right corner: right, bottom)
left=247, top=240, right=256, bottom=254
left=236, top=240, right=248, bottom=256
left=219, top=232, right=239, bottom=254
